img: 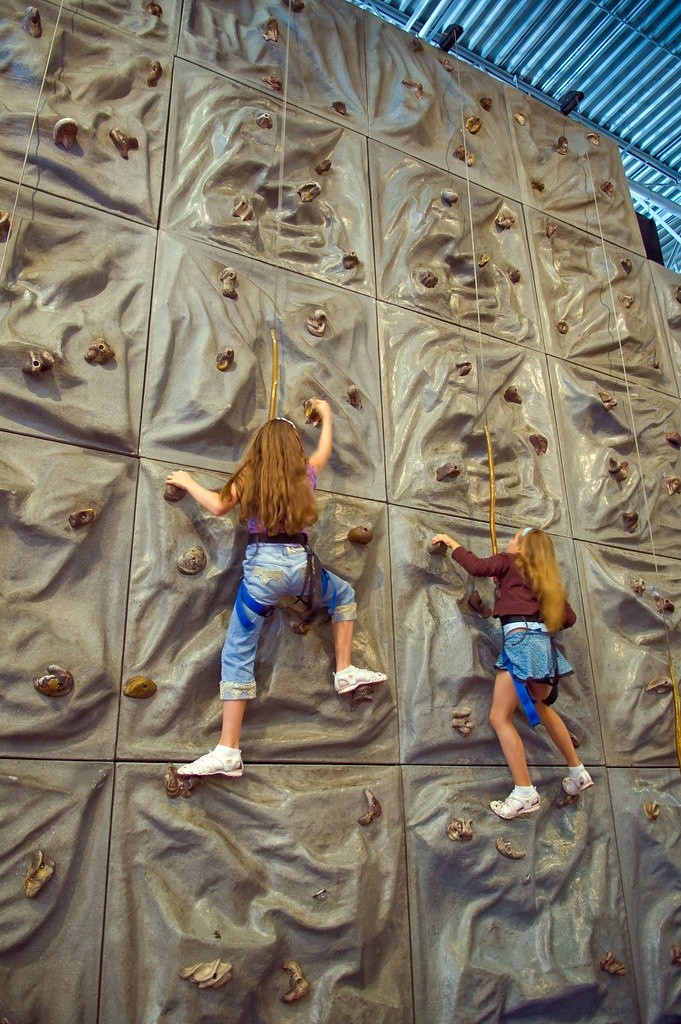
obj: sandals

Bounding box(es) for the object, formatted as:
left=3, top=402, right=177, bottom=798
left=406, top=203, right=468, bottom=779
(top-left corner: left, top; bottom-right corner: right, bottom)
left=490, top=788, right=540, bottom=819
left=562, top=768, right=595, bottom=795
left=175, top=747, right=244, bottom=777
left=333, top=665, right=387, bottom=695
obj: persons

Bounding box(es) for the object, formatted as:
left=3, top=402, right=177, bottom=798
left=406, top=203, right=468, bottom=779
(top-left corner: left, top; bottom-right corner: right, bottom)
left=164, top=400, right=387, bottom=778
left=433, top=526, right=595, bottom=820
